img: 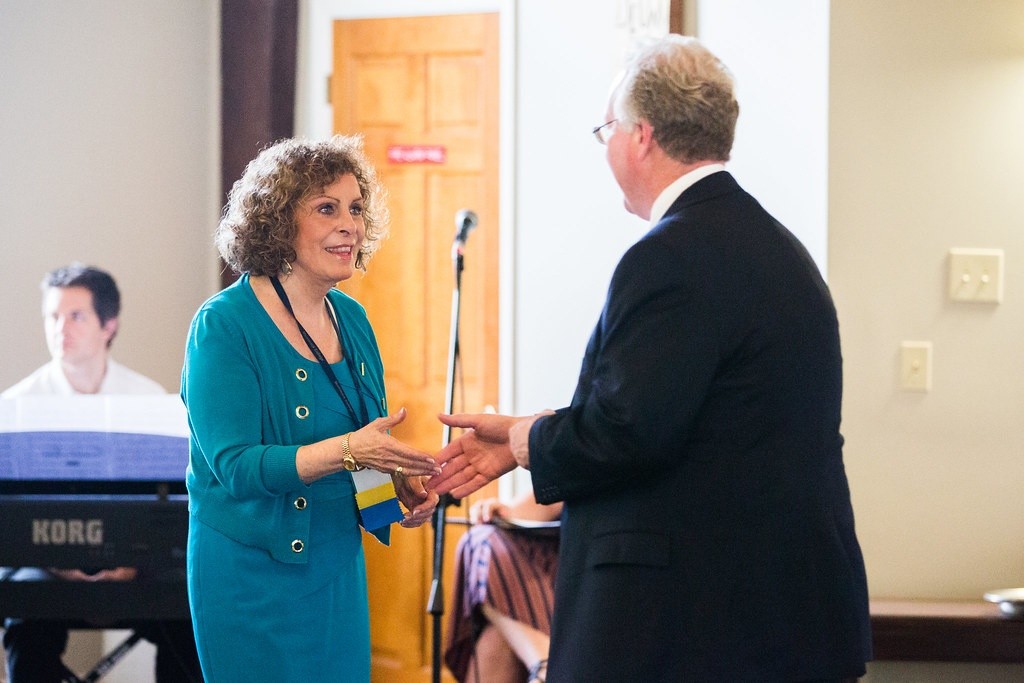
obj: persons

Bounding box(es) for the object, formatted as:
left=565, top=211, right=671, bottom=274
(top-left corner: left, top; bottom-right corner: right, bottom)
left=179, top=133, right=442, bottom=683
left=0, top=263, right=205, bottom=683
left=426, top=33, right=874, bottom=683
left=449, top=493, right=563, bottom=683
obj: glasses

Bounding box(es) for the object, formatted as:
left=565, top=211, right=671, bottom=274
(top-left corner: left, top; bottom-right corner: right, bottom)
left=593, top=118, right=642, bottom=145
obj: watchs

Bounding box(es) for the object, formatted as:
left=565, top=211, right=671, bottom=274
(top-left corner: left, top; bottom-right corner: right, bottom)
left=342, top=431, right=356, bottom=471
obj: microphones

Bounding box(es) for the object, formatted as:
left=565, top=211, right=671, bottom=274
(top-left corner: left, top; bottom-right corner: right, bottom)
left=452, top=209, right=478, bottom=253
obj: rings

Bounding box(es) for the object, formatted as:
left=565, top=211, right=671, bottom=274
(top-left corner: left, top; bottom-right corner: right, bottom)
left=394, top=467, right=404, bottom=479
